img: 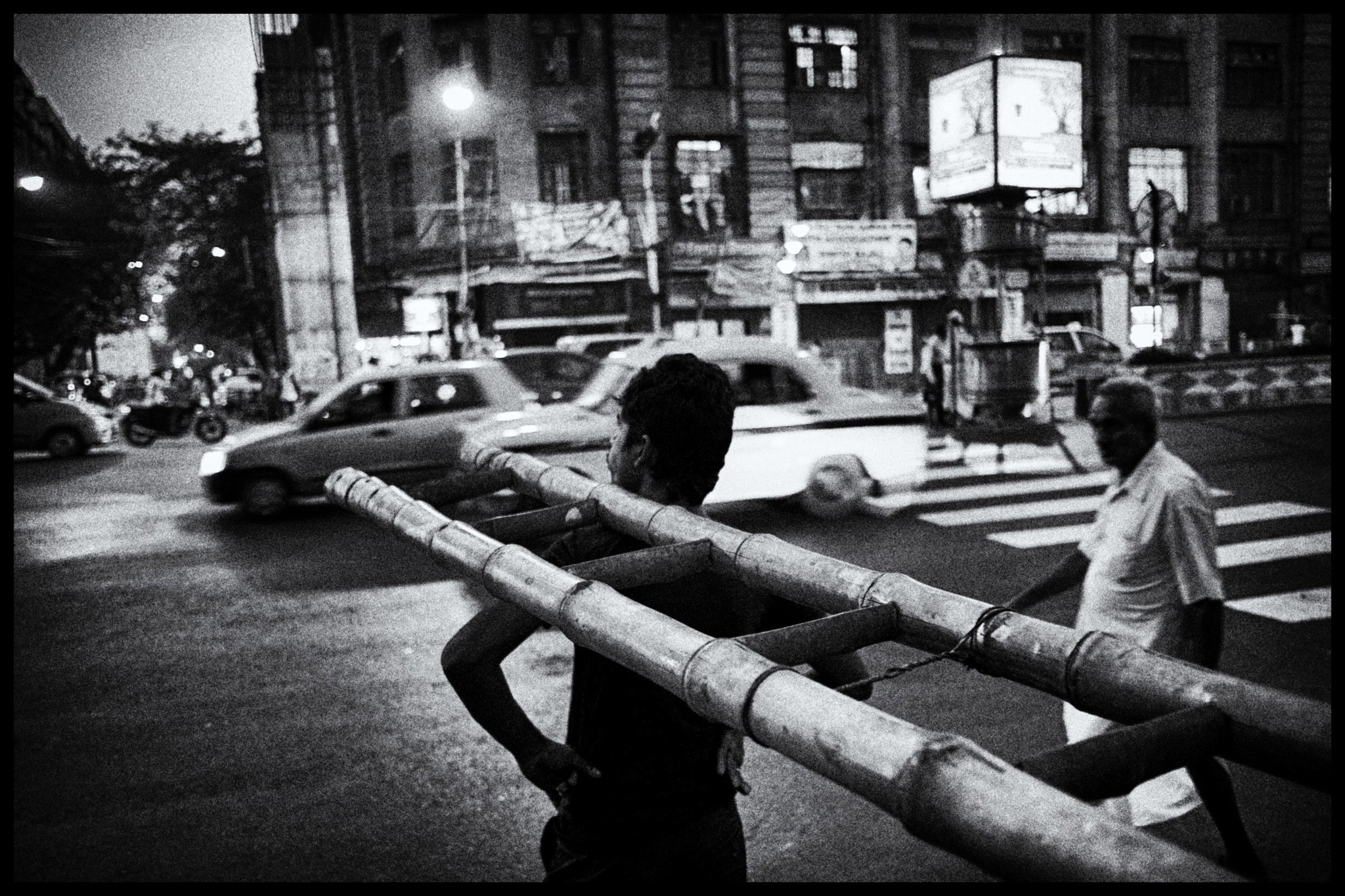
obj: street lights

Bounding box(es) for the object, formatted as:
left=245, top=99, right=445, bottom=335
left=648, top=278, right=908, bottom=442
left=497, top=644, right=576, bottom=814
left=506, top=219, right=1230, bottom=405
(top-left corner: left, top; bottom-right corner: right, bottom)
left=437, top=72, right=480, bottom=360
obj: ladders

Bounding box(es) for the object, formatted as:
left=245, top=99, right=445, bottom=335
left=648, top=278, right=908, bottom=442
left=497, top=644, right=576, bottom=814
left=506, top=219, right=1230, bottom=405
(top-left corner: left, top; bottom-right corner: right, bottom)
left=323, top=439, right=1335, bottom=888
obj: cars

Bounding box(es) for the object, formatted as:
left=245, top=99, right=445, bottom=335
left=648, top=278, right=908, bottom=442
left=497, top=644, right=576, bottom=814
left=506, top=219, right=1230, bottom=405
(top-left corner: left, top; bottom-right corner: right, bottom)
left=192, top=359, right=537, bottom=525
left=458, top=337, right=933, bottom=523
left=1039, top=327, right=1130, bottom=389
left=480, top=345, right=604, bottom=408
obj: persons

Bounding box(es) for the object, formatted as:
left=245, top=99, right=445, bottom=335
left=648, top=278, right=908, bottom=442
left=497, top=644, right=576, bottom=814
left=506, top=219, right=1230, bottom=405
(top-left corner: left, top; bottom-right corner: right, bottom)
left=144, top=361, right=300, bottom=422
left=439, top=353, right=873, bottom=896
left=1001, top=376, right=1230, bottom=828
left=915, top=310, right=972, bottom=430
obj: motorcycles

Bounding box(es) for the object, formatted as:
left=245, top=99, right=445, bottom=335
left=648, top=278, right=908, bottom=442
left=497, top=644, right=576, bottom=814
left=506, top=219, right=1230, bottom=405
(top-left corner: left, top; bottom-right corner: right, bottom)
left=118, top=398, right=227, bottom=449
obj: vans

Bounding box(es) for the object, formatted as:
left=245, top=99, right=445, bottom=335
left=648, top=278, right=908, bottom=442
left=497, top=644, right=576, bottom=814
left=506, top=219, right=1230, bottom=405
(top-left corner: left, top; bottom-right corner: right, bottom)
left=11, top=370, right=111, bottom=465
